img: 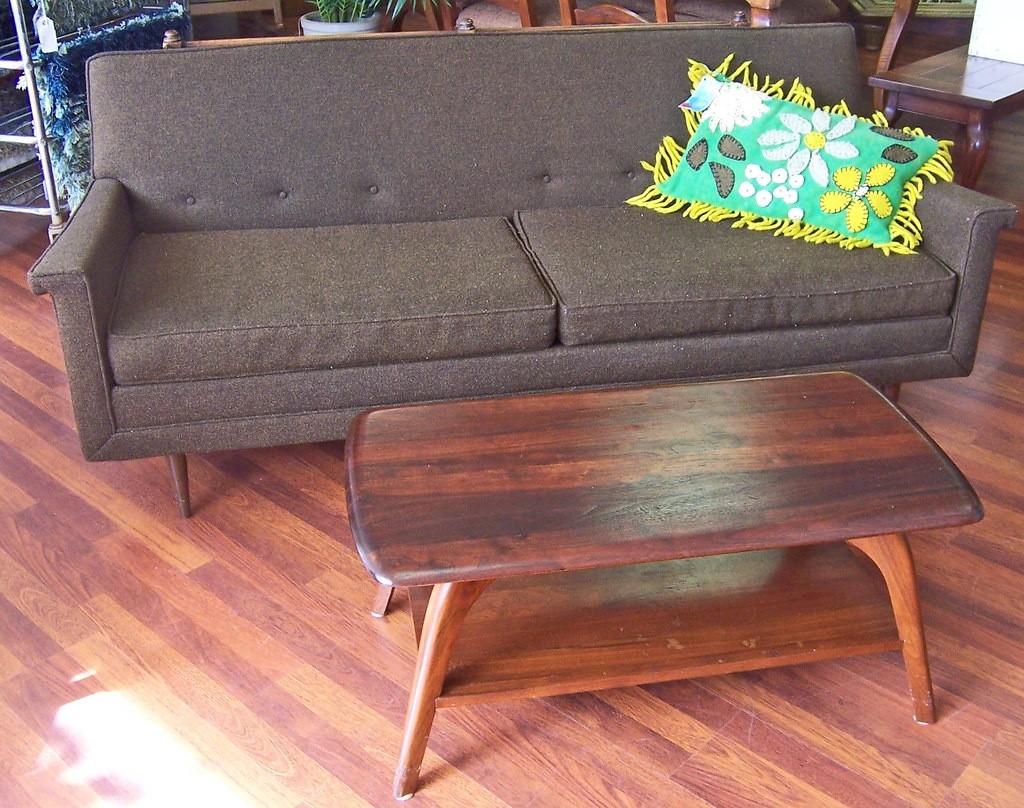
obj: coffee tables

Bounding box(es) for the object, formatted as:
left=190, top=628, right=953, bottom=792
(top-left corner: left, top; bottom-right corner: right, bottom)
left=346, top=371, right=984, bottom=801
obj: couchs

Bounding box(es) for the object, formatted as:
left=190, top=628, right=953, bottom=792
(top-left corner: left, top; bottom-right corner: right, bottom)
left=27, top=22, right=1017, bottom=517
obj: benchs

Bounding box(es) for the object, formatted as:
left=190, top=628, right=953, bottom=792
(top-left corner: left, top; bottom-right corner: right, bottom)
left=868, top=44, right=1024, bottom=190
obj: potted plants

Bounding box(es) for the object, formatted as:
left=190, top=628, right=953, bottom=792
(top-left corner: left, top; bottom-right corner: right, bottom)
left=300, top=0, right=452, bottom=37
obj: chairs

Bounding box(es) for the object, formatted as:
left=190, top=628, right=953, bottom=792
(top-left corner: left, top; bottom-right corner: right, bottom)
left=423, top=0, right=593, bottom=28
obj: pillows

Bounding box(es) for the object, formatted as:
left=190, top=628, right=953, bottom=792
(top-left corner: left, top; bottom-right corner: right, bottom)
left=623, top=53, right=954, bottom=256
